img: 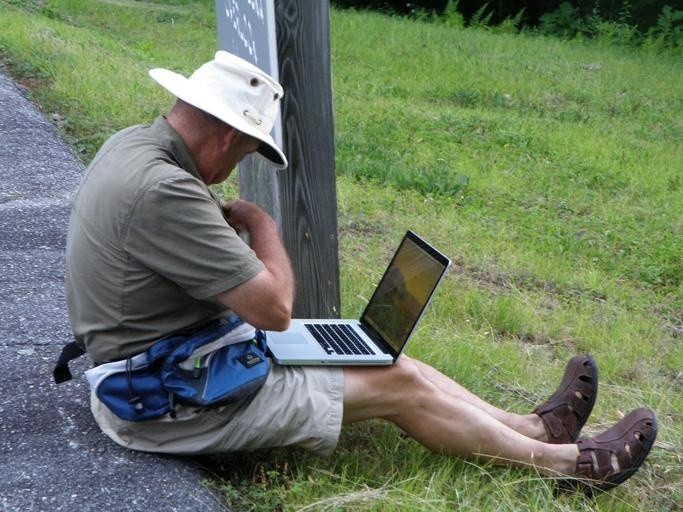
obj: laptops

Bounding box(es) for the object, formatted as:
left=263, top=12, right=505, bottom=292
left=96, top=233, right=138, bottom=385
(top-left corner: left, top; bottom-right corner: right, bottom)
left=263, top=227, right=452, bottom=367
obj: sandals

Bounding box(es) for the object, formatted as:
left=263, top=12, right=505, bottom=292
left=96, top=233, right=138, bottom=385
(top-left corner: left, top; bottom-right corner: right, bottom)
left=553, top=407, right=657, bottom=501
left=532, top=355, right=598, bottom=445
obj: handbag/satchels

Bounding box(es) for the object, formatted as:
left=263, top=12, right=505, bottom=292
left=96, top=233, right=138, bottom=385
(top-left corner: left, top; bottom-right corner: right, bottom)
left=85, top=312, right=268, bottom=423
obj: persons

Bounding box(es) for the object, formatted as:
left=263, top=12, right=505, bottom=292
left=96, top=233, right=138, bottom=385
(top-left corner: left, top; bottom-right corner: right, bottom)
left=58, top=49, right=657, bottom=500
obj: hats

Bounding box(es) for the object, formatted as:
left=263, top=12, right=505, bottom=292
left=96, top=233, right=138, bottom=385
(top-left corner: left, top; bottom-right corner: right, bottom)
left=148, top=50, right=288, bottom=171
